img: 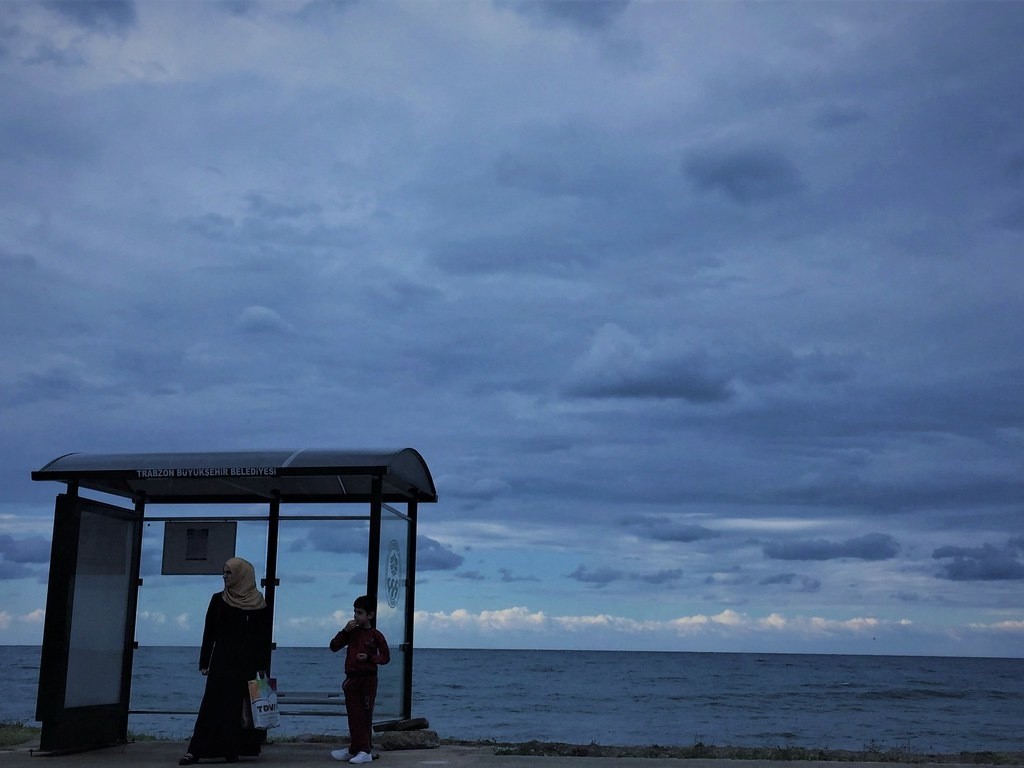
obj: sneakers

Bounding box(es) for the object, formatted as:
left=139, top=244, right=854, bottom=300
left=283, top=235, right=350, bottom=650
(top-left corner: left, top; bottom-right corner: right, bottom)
left=331, top=747, right=355, bottom=760
left=350, top=751, right=372, bottom=764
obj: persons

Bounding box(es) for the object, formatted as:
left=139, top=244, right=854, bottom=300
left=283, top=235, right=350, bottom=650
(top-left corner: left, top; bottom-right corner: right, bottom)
left=179, top=556, right=273, bottom=766
left=329, top=595, right=390, bottom=764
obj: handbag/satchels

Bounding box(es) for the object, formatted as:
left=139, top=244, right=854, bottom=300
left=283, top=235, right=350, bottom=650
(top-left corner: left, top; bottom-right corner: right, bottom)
left=248, top=671, right=280, bottom=730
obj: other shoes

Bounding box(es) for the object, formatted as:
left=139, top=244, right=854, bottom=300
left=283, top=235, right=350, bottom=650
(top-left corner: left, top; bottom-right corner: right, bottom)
left=225, top=756, right=239, bottom=763
left=179, top=752, right=199, bottom=764
